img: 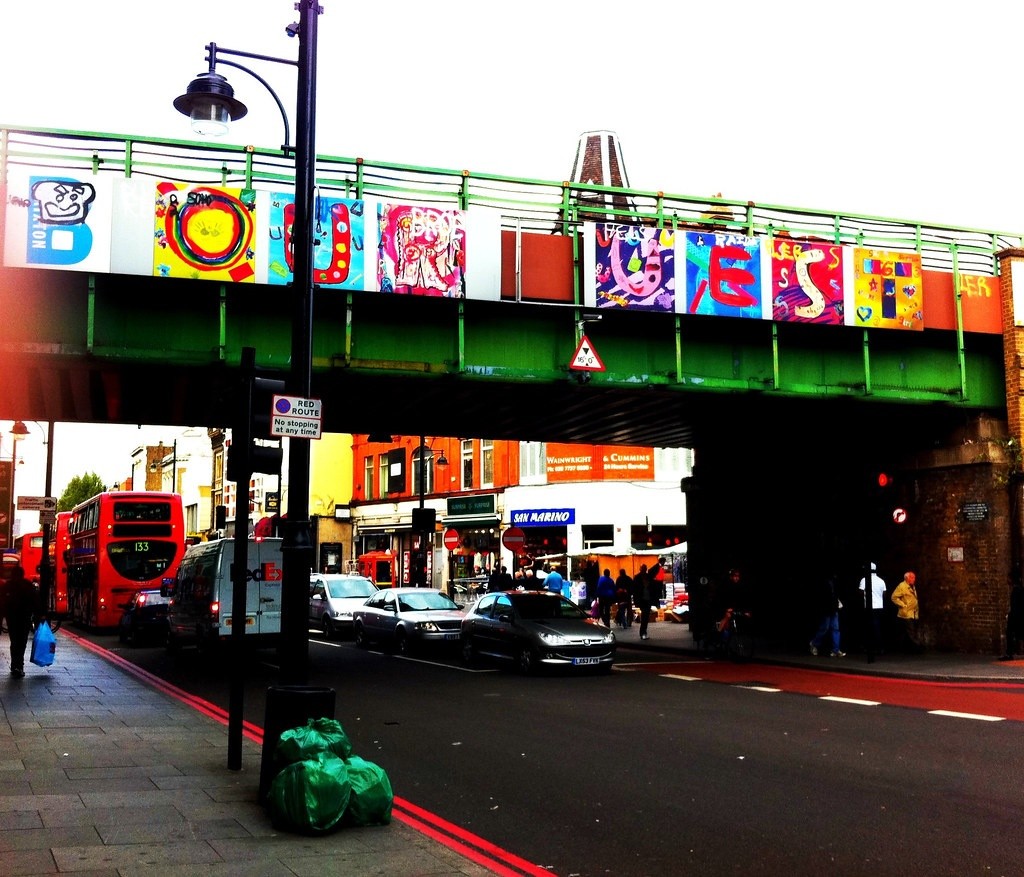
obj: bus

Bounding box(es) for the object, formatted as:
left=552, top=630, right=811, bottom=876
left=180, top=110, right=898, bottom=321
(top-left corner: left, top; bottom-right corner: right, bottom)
left=14, top=532, right=43, bottom=584
left=62, top=490, right=185, bottom=626
left=36, top=512, right=72, bottom=613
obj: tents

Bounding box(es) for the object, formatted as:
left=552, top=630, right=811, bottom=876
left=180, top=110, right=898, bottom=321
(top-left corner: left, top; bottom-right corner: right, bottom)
left=535, top=540, right=688, bottom=596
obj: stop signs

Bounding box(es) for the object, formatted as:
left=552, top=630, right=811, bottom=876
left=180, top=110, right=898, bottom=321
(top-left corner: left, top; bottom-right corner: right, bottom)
left=502, top=527, right=525, bottom=552
left=444, top=529, right=459, bottom=551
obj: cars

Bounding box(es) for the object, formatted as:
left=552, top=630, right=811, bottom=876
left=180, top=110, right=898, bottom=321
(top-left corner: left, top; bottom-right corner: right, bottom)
left=462, top=575, right=491, bottom=595
left=460, top=590, right=616, bottom=673
left=353, top=587, right=466, bottom=656
left=116, top=589, right=173, bottom=644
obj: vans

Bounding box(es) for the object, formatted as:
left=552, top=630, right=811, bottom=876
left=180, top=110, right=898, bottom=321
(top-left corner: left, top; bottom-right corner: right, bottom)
left=160, top=537, right=282, bottom=659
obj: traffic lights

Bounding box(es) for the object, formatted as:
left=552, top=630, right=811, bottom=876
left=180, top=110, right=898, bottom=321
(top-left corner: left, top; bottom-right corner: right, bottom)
left=226, top=347, right=285, bottom=483
left=216, top=506, right=226, bottom=531
left=412, top=508, right=436, bottom=533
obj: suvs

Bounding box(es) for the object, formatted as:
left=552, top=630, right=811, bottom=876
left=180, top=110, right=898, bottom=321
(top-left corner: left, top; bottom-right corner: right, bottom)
left=310, top=573, right=380, bottom=639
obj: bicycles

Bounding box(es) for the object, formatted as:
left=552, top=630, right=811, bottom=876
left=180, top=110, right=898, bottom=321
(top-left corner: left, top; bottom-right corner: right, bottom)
left=696, top=609, right=755, bottom=663
left=1, top=610, right=62, bottom=634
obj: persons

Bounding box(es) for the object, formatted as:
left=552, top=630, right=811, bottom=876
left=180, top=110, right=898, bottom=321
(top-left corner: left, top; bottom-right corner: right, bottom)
left=713, top=568, right=751, bottom=659
left=489, top=558, right=666, bottom=640
left=996, top=571, right=1024, bottom=661
left=859, top=562, right=887, bottom=658
left=0, top=566, right=46, bottom=676
left=890, top=571, right=927, bottom=654
left=809, top=568, right=847, bottom=658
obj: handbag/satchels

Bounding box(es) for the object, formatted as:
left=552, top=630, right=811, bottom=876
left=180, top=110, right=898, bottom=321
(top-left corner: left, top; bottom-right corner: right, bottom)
left=590, top=600, right=602, bottom=619
left=30, top=621, right=55, bottom=667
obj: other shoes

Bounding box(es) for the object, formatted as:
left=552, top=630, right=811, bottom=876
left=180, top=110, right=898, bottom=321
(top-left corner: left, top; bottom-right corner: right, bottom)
left=809, top=641, right=817, bottom=655
left=10, top=667, right=25, bottom=678
left=641, top=634, right=650, bottom=640
left=830, top=650, right=846, bottom=657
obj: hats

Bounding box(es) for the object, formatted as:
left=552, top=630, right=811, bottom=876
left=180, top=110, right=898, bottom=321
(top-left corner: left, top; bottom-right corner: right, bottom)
left=863, top=562, right=876, bottom=570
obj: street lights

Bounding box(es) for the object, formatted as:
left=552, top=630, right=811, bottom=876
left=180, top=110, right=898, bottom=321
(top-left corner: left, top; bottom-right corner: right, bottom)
left=150, top=460, right=175, bottom=494
left=11, top=420, right=54, bottom=616
left=174, top=1, right=322, bottom=685
left=417, top=448, right=449, bottom=587
